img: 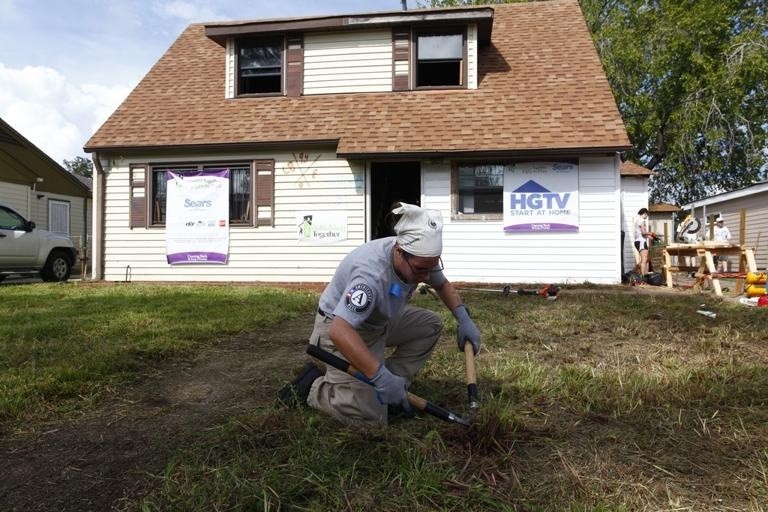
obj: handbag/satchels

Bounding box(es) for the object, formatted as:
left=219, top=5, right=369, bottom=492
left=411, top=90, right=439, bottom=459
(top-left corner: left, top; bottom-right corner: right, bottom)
left=644, top=271, right=664, bottom=287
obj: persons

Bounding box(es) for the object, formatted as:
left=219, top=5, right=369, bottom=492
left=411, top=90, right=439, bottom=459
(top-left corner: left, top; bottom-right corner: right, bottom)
left=704, top=215, right=733, bottom=274
left=634, top=206, right=657, bottom=278
left=274, top=197, right=483, bottom=439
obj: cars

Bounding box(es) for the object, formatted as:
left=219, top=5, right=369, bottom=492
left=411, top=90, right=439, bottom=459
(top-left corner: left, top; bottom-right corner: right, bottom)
left=0, top=205, right=76, bottom=282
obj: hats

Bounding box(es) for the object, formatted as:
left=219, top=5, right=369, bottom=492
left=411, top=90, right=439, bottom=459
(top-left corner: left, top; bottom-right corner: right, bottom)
left=715, top=217, right=725, bottom=223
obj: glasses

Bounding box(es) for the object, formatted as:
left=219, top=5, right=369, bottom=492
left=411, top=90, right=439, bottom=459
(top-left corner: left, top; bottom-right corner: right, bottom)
left=402, top=252, right=444, bottom=275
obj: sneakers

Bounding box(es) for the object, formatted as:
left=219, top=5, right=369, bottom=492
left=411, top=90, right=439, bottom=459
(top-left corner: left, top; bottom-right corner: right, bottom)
left=275, top=361, right=326, bottom=410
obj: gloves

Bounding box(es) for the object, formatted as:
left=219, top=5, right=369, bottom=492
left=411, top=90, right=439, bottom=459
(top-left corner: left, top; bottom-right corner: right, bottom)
left=369, top=364, right=414, bottom=417
left=453, top=303, right=480, bottom=356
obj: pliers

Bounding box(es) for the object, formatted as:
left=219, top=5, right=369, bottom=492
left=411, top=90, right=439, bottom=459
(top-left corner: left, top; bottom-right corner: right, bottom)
left=307, top=306, right=482, bottom=443
